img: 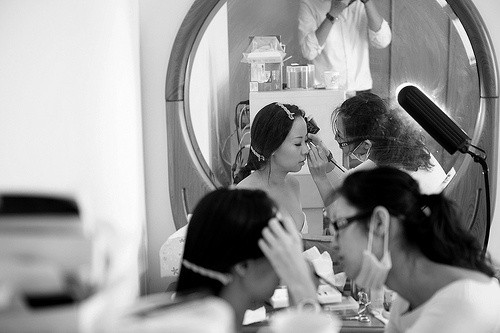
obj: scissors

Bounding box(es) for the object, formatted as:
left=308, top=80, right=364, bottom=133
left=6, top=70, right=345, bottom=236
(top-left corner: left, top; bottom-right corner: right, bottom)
left=343, top=315, right=370, bottom=322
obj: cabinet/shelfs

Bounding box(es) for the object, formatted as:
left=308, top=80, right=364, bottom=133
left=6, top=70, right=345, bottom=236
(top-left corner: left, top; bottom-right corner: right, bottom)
left=250, top=86, right=346, bottom=175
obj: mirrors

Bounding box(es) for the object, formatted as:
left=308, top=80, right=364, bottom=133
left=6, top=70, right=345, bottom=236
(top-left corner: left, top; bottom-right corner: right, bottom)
left=164, top=0, right=500, bottom=290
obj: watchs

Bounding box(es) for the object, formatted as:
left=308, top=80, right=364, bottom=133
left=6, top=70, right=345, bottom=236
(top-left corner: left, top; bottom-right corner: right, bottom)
left=295, top=299, right=325, bottom=316
left=326, top=150, right=333, bottom=162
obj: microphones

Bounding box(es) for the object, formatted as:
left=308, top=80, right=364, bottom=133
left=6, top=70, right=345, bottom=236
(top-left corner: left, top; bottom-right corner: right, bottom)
left=397, top=86, right=469, bottom=155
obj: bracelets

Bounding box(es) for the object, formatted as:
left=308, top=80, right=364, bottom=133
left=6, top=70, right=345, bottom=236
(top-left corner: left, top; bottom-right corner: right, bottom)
left=326, top=12, right=337, bottom=23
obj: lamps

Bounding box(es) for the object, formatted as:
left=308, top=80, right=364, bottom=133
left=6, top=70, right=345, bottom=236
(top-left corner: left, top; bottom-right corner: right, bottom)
left=396, top=81, right=491, bottom=259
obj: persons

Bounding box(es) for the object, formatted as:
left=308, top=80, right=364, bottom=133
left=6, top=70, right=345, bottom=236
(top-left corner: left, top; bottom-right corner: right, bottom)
left=258, top=165, right=500, bottom=333
left=298, top=0, right=392, bottom=100
left=117, top=186, right=287, bottom=333
left=307, top=92, right=447, bottom=237
left=233, top=102, right=310, bottom=234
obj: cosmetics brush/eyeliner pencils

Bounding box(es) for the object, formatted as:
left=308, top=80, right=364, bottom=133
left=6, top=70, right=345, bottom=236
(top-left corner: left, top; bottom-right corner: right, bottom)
left=331, top=159, right=345, bottom=173
left=314, top=271, right=350, bottom=299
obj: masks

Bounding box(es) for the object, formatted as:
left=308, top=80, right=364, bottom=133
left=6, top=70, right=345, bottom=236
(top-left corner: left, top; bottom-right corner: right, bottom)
left=352, top=205, right=393, bottom=292
left=342, top=137, right=374, bottom=170
